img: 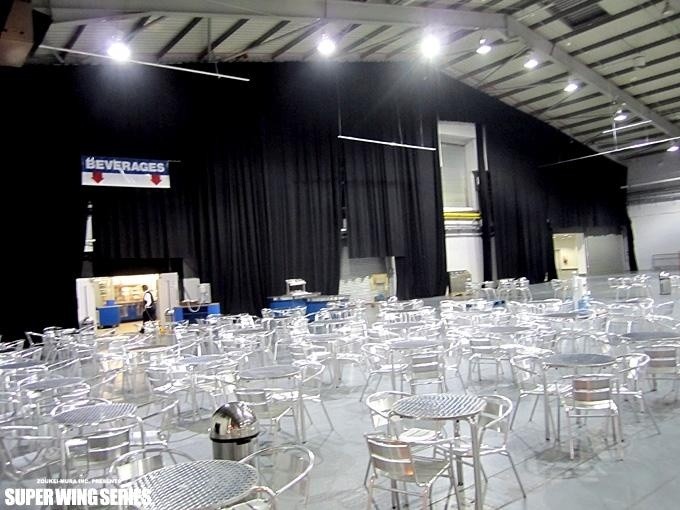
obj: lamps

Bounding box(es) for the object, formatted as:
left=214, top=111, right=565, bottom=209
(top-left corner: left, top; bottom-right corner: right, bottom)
left=563, top=81, right=578, bottom=92
left=613, top=109, right=627, bottom=121
left=523, top=55, right=538, bottom=68
left=316, top=29, right=335, bottom=55
left=666, top=140, right=680, bottom=153
left=476, top=33, right=492, bottom=55
left=662, top=1, right=674, bottom=16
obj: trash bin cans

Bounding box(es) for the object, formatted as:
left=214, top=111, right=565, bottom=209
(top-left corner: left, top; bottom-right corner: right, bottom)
left=660, top=276, right=672, bottom=295
left=210, top=401, right=260, bottom=461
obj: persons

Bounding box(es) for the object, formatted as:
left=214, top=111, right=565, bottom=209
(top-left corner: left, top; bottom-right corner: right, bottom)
left=138, top=284, right=157, bottom=334
